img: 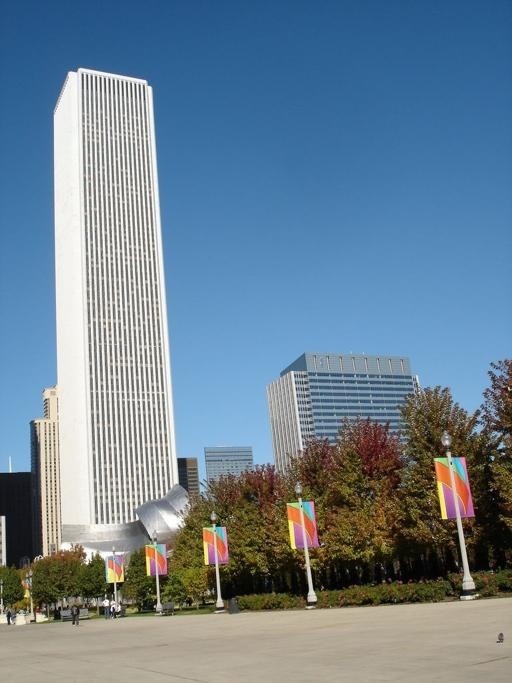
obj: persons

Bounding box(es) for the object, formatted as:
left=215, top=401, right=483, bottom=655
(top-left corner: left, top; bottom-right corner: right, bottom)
left=5, top=609, right=11, bottom=624
left=102, top=597, right=110, bottom=619
left=70, top=602, right=79, bottom=625
left=110, top=599, right=120, bottom=619
left=24, top=605, right=61, bottom=619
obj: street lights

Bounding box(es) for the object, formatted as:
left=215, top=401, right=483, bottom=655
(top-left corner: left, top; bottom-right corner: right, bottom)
left=293, top=482, right=320, bottom=609
left=109, top=545, right=120, bottom=603
left=438, top=427, right=483, bottom=599
left=209, top=511, right=227, bottom=613
left=25, top=568, right=34, bottom=615
left=152, top=530, right=166, bottom=614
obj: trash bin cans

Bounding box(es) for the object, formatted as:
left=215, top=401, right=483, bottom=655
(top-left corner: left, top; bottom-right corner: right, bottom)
left=229, top=597, right=239, bottom=614
left=54, top=610, right=60, bottom=620
